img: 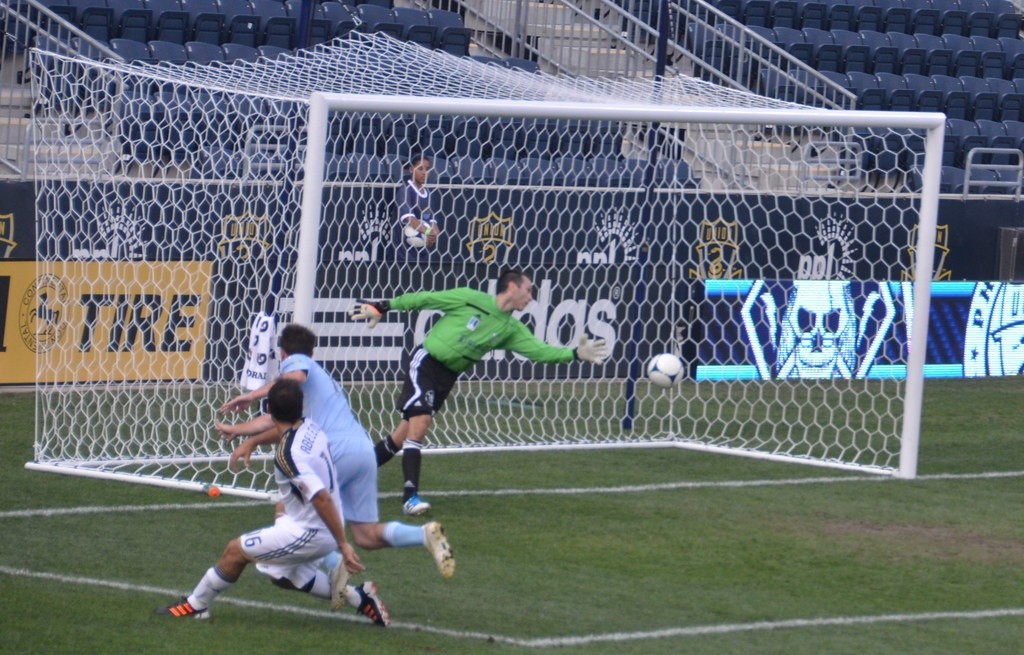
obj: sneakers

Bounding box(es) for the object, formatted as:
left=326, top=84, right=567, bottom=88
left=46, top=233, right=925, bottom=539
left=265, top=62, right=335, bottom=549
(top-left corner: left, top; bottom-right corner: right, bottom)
left=421, top=522, right=455, bottom=578
left=155, top=596, right=214, bottom=623
left=329, top=556, right=352, bottom=611
left=354, top=580, right=389, bottom=627
left=403, top=496, right=430, bottom=516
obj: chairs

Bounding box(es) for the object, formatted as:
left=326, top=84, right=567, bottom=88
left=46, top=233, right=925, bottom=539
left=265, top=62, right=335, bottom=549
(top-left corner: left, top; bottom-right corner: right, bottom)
left=0, top=0, right=1024, bottom=198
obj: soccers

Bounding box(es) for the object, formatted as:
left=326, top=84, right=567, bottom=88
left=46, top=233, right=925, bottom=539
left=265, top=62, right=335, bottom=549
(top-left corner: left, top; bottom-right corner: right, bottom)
left=646, top=353, right=686, bottom=388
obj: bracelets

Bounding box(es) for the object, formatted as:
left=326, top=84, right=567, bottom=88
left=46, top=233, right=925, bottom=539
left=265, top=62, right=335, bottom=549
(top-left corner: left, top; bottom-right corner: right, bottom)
left=425, top=228, right=431, bottom=234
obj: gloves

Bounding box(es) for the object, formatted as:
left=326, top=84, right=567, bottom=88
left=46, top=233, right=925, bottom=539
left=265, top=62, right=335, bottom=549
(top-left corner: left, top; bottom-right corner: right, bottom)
left=348, top=298, right=390, bottom=328
left=576, top=334, right=607, bottom=365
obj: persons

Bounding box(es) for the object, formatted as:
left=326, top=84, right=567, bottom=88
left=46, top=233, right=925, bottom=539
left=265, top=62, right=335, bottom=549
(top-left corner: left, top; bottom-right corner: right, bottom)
left=220, top=324, right=455, bottom=612
left=154, top=380, right=389, bottom=627
left=391, top=155, right=441, bottom=261
left=351, top=271, right=608, bottom=517
